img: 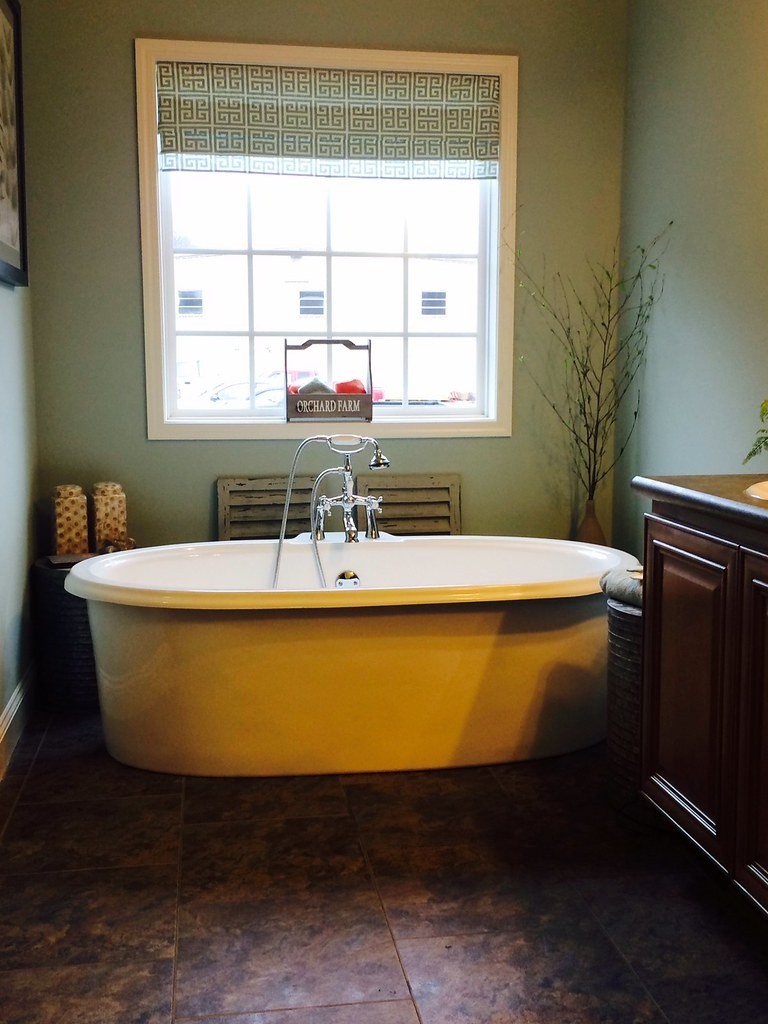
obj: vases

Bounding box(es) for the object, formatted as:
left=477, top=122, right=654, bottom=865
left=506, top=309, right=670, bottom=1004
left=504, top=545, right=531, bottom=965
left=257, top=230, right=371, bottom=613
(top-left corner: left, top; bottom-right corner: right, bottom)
left=578, top=499, right=606, bottom=546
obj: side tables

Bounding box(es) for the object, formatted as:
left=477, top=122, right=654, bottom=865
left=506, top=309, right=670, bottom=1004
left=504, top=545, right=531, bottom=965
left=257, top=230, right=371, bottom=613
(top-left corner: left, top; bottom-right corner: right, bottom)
left=601, top=599, right=643, bottom=823
left=32, top=562, right=99, bottom=714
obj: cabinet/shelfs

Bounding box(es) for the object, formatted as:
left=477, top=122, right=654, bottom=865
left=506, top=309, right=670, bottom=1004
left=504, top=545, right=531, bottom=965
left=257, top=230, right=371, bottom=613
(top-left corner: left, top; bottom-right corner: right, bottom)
left=637, top=497, right=768, bottom=918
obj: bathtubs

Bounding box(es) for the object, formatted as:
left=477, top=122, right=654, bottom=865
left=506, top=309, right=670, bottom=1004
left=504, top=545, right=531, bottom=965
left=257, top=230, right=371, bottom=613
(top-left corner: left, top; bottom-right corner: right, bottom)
left=65, top=534, right=643, bottom=777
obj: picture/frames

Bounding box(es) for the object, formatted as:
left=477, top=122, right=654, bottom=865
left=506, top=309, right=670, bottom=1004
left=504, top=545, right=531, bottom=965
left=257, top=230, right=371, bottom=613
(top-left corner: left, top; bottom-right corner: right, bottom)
left=0, top=0, right=28, bottom=288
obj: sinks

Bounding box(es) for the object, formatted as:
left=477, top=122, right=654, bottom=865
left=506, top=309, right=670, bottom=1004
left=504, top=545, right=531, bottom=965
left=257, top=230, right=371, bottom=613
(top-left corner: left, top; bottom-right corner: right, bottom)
left=744, top=481, right=768, bottom=500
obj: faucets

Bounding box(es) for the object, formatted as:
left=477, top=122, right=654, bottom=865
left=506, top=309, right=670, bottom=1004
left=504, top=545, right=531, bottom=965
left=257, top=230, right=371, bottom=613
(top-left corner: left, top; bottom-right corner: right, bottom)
left=308, top=436, right=390, bottom=539
